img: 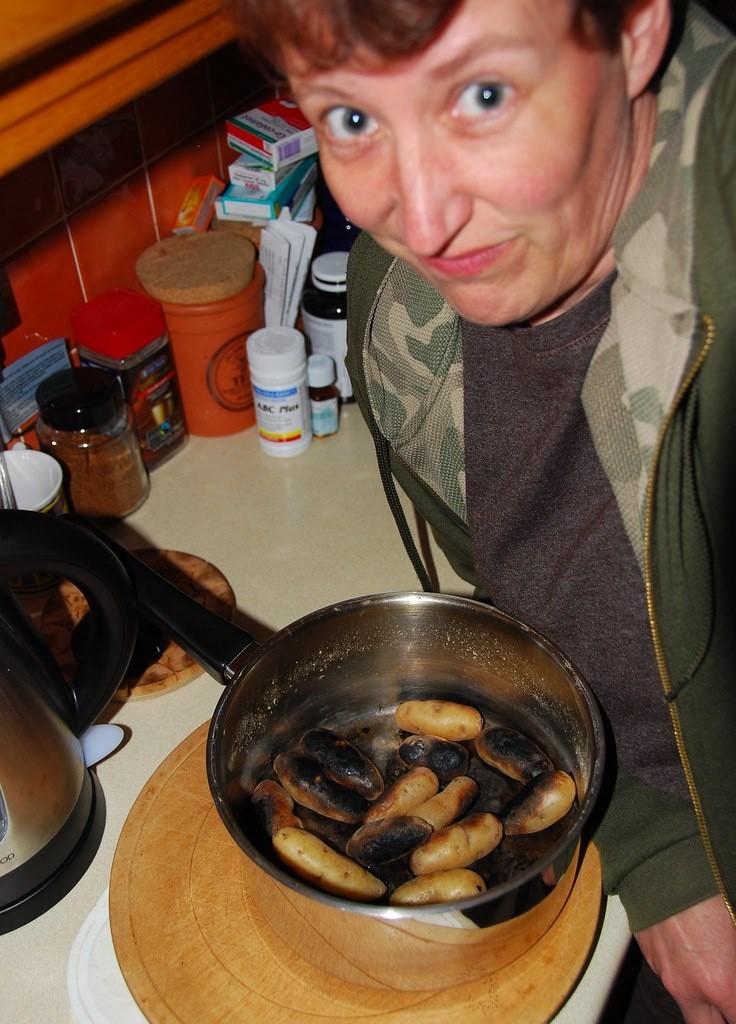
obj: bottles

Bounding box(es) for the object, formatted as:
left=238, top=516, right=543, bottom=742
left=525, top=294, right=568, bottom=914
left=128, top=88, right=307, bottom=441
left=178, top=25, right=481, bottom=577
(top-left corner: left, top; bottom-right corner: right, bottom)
left=245, top=248, right=353, bottom=459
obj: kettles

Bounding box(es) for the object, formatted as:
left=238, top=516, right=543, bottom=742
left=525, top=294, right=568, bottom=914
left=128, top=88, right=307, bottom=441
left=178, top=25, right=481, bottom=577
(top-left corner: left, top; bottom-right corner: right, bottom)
left=0, top=508, right=139, bottom=937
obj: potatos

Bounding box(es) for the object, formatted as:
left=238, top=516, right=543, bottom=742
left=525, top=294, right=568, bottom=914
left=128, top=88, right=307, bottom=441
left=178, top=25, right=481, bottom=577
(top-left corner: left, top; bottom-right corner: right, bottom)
left=248, top=699, right=575, bottom=905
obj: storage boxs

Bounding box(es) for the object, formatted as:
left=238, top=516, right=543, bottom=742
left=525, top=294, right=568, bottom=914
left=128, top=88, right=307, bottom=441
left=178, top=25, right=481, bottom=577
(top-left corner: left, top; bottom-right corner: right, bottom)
left=228, top=152, right=296, bottom=191
left=226, top=98, right=319, bottom=171
left=220, top=156, right=314, bottom=221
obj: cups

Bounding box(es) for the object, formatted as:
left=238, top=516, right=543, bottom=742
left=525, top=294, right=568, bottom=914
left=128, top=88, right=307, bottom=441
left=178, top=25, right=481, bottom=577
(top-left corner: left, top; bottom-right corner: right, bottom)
left=0, top=450, right=68, bottom=515
left=160, top=265, right=266, bottom=438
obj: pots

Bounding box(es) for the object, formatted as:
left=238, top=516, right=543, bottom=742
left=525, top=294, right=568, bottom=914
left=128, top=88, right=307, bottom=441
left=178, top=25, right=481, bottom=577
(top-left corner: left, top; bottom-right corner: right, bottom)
left=108, top=540, right=623, bottom=990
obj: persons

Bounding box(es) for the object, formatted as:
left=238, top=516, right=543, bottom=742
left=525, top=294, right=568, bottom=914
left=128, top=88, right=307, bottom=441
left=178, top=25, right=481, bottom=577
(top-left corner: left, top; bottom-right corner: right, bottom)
left=229, top=0, right=736, bottom=1024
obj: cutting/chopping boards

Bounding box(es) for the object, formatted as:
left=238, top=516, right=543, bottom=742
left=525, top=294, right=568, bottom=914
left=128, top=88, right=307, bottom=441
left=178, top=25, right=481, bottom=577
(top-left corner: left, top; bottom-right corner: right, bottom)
left=108, top=718, right=601, bottom=1024
